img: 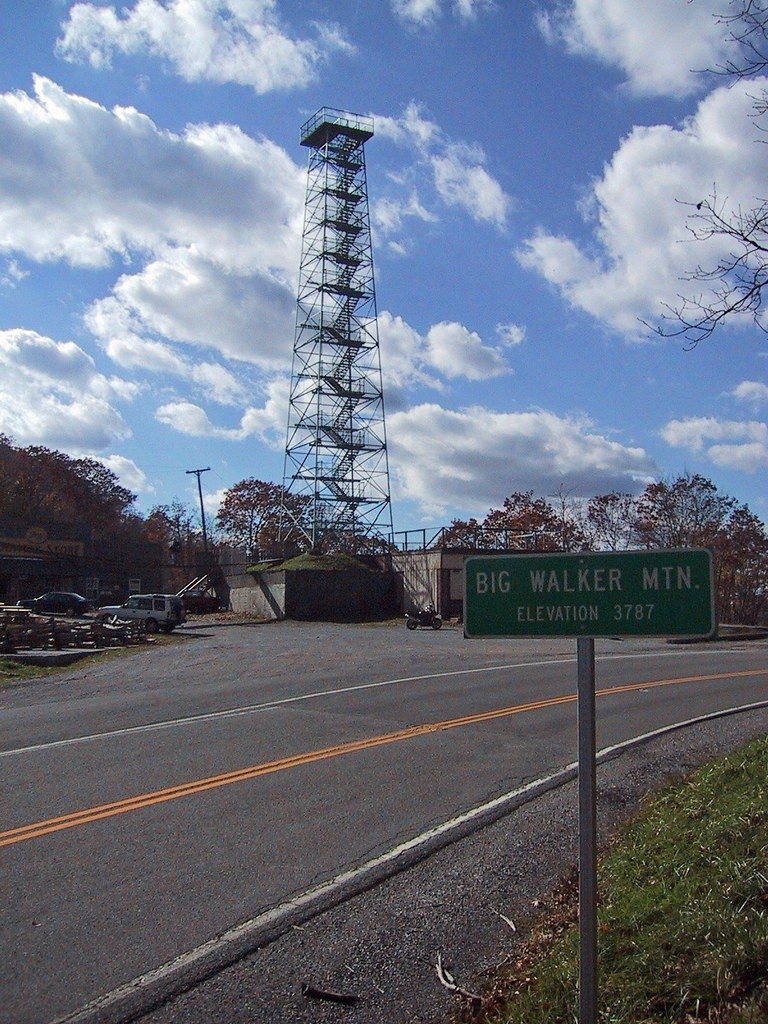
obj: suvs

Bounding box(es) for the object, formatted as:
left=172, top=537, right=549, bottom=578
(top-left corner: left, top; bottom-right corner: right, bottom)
left=98, top=594, right=186, bottom=633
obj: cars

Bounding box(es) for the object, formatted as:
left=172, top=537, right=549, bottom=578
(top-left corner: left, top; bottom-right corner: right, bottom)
left=16, top=591, right=92, bottom=617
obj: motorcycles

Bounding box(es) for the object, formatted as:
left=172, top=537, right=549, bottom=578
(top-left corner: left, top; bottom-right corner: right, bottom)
left=404, top=605, right=442, bottom=629
